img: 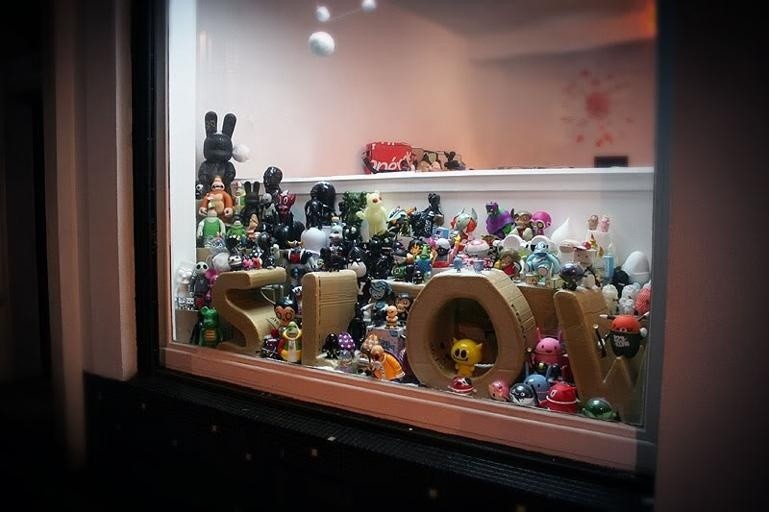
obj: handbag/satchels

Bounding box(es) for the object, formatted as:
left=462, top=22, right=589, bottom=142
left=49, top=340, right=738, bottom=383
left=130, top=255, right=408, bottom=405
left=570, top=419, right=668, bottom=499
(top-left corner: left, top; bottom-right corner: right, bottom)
left=362, top=141, right=415, bottom=175
